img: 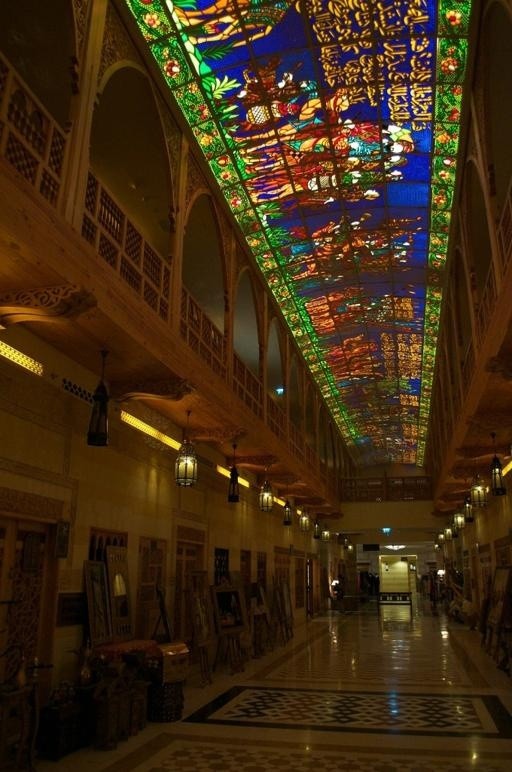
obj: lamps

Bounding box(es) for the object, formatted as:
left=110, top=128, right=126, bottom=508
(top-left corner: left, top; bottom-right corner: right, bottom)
left=490, top=432, right=506, bottom=496
left=322, top=530, right=330, bottom=542
left=444, top=528, right=454, bottom=541
left=470, top=472, right=491, bottom=509
left=87, top=350, right=111, bottom=448
left=437, top=533, right=444, bottom=544
left=299, top=512, right=310, bottom=533
left=434, top=544, right=439, bottom=553
left=463, top=496, right=473, bottom=523
left=452, top=513, right=466, bottom=539
left=175, top=408, right=198, bottom=488
left=283, top=502, right=292, bottom=526
left=313, top=521, right=321, bottom=539
left=229, top=442, right=240, bottom=503
left=258, top=479, right=275, bottom=513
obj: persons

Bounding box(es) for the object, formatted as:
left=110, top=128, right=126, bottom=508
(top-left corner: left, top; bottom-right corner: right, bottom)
left=334, top=575, right=351, bottom=613
